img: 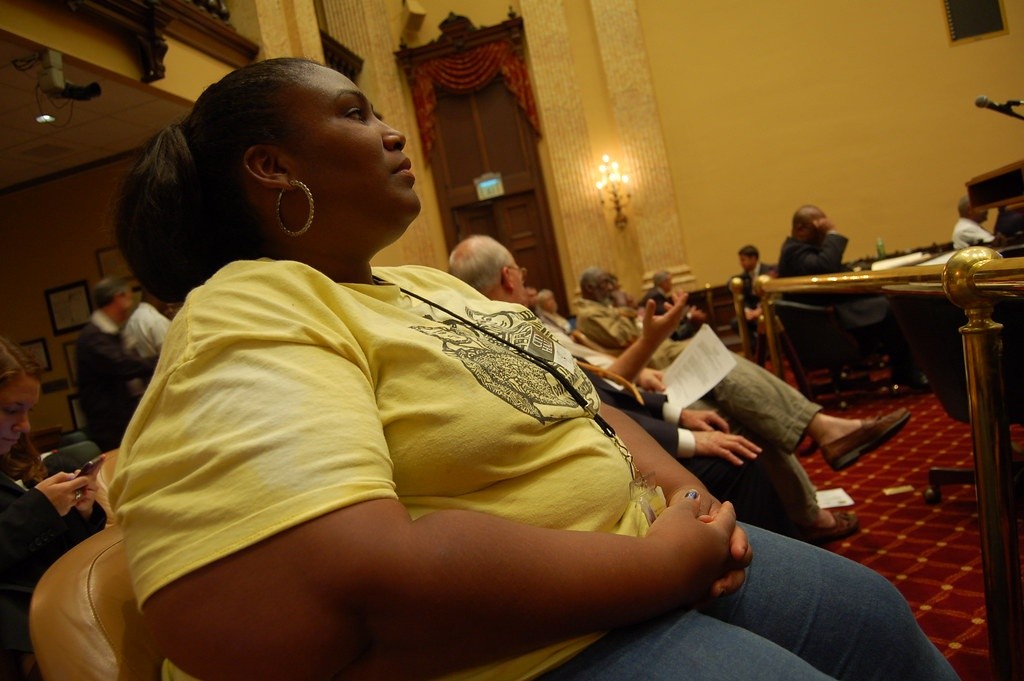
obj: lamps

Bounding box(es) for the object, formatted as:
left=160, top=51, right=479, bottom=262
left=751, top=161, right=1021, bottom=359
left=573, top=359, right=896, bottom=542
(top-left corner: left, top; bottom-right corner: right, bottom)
left=595, top=154, right=634, bottom=230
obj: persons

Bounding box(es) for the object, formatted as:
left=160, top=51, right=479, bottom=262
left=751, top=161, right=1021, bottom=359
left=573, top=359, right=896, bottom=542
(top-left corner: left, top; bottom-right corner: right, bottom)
left=71, top=263, right=180, bottom=452
left=952, top=195, right=1005, bottom=250
left=448, top=234, right=910, bottom=543
left=110, top=56, right=962, bottom=681
left=0, top=336, right=107, bottom=681
left=777, top=204, right=925, bottom=389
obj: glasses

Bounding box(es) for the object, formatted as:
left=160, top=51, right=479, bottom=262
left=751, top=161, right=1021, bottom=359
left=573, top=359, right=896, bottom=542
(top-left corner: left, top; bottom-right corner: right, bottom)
left=501, top=265, right=528, bottom=287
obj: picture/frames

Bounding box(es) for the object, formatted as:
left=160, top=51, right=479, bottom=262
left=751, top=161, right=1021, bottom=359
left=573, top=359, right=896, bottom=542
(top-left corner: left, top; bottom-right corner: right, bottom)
left=44, top=279, right=93, bottom=336
left=67, top=394, right=88, bottom=431
left=21, top=337, right=52, bottom=374
left=95, top=245, right=137, bottom=281
left=63, top=340, right=79, bottom=386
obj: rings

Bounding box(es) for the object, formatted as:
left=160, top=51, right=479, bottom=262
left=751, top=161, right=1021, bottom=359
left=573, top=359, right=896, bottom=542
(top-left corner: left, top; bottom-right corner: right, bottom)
left=76, top=491, right=81, bottom=500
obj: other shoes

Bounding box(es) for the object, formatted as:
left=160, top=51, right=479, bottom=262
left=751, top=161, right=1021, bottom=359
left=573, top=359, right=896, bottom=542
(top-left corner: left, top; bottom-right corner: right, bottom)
left=792, top=513, right=860, bottom=545
left=823, top=406, right=911, bottom=472
left=910, top=382, right=935, bottom=396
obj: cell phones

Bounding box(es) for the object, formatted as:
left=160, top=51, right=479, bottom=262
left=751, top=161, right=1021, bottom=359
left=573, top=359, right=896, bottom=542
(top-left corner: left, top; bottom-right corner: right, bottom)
left=75, top=454, right=106, bottom=478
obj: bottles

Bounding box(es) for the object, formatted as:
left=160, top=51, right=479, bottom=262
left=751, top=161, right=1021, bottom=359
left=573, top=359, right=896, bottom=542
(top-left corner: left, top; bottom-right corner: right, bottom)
left=876, top=238, right=887, bottom=259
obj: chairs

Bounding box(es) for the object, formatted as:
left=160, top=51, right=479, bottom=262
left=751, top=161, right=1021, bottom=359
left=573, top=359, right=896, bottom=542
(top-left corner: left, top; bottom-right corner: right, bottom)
left=29, top=526, right=168, bottom=681
left=776, top=294, right=1024, bottom=504
left=572, top=330, right=624, bottom=357
left=93, top=446, right=119, bottom=527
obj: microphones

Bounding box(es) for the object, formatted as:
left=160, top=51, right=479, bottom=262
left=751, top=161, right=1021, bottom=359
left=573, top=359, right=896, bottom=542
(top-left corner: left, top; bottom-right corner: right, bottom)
left=975, top=95, right=1024, bottom=120
